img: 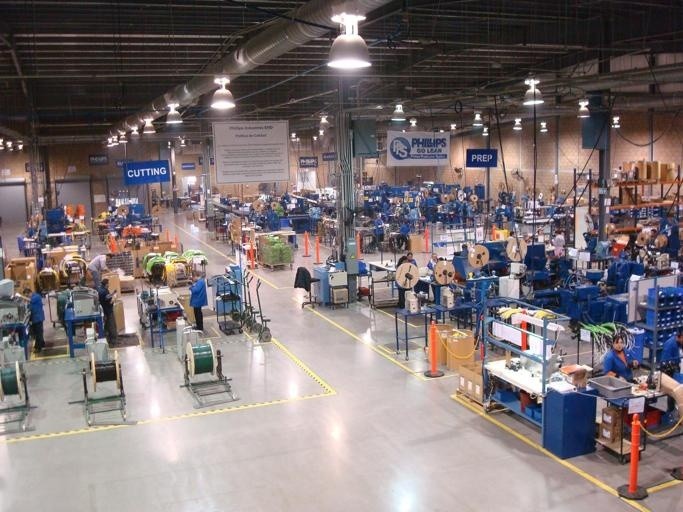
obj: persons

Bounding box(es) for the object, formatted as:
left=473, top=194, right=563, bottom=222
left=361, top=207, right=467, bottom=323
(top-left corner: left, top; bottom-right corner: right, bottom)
left=87, top=253, right=113, bottom=292
left=99, top=278, right=121, bottom=344
left=23, top=287, right=46, bottom=353
left=602, top=334, right=640, bottom=383
left=187, top=271, right=208, bottom=330
left=226, top=193, right=676, bottom=307
left=656, top=327, right=683, bottom=384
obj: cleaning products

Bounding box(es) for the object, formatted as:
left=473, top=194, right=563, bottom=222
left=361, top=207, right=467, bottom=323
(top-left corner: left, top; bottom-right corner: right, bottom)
left=240, top=268, right=271, bottom=342
left=215, top=277, right=243, bottom=336
left=356, top=271, right=372, bottom=302
left=593, top=393, right=648, bottom=464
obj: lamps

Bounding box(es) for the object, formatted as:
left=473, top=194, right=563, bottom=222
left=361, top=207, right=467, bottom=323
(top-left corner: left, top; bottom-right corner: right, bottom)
left=166, top=104, right=183, bottom=123
left=211, top=78, right=236, bottom=109
left=106, top=138, right=114, bottom=148
left=143, top=119, right=156, bottom=134
left=289, top=76, right=622, bottom=152
left=131, top=126, right=139, bottom=136
left=111, top=135, right=119, bottom=146
left=119, top=132, right=128, bottom=143
left=1, top=137, right=27, bottom=152
left=327, top=14, right=371, bottom=69
